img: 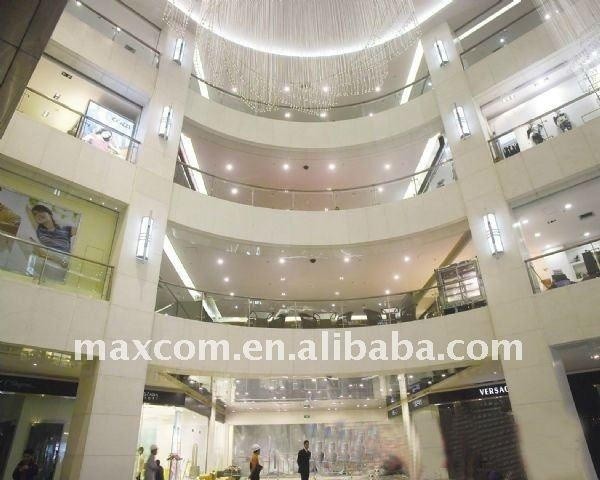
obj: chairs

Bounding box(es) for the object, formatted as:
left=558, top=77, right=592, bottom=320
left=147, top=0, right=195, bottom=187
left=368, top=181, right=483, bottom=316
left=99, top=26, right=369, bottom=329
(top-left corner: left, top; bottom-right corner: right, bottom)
left=247, top=298, right=485, bottom=328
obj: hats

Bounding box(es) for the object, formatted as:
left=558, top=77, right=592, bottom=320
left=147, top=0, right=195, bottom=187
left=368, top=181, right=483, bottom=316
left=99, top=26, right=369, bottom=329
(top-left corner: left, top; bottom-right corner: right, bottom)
left=151, top=444, right=158, bottom=451
left=251, top=444, right=261, bottom=453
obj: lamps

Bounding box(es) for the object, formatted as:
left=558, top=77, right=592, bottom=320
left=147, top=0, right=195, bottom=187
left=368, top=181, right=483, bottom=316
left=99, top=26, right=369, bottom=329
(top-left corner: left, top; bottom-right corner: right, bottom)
left=137, top=216, right=153, bottom=262
left=484, top=213, right=504, bottom=257
left=159, top=106, right=173, bottom=139
left=174, top=39, right=185, bottom=65
left=452, top=106, right=470, bottom=139
left=433, top=40, right=448, bottom=67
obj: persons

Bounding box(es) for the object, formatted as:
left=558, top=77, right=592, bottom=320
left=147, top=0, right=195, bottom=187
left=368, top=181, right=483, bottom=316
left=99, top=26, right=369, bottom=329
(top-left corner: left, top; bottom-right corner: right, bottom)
left=527, top=120, right=545, bottom=144
left=133, top=447, right=163, bottom=480
left=553, top=110, right=571, bottom=132
left=26, top=205, right=72, bottom=284
left=82, top=128, right=120, bottom=156
left=250, top=445, right=261, bottom=480
left=297, top=440, right=311, bottom=480
left=12, top=449, right=39, bottom=480
